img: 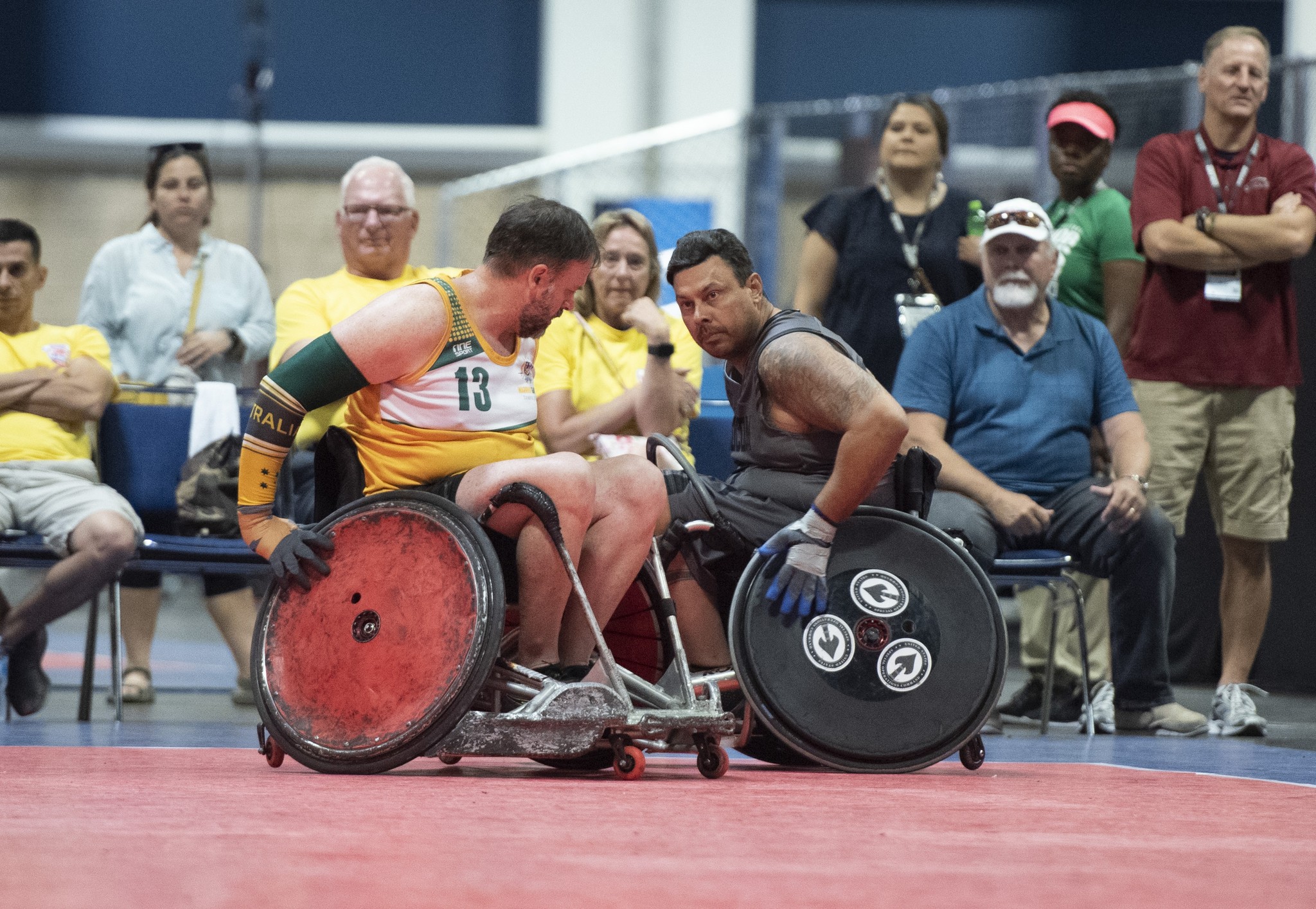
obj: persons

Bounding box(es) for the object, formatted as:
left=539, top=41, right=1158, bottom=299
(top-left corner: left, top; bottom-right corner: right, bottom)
left=793, top=90, right=996, bottom=520
left=76, top=142, right=275, bottom=707
left=265, top=156, right=474, bottom=529
left=0, top=214, right=146, bottom=715
left=655, top=228, right=911, bottom=751
left=533, top=210, right=708, bottom=749
left=891, top=197, right=1209, bottom=738
left=236, top=198, right=672, bottom=772
left=958, top=90, right=1145, bottom=727
left=1079, top=25, right=1316, bottom=738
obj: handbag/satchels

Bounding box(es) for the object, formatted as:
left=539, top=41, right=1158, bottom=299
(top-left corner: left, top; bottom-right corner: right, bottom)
left=174, top=432, right=245, bottom=538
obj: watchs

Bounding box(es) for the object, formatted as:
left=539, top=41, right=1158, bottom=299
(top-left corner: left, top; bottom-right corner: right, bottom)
left=648, top=342, right=674, bottom=359
left=1117, top=472, right=1151, bottom=495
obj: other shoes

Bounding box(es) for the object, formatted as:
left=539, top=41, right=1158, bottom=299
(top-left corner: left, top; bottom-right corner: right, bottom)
left=232, top=676, right=258, bottom=706
left=6, top=624, right=50, bottom=717
left=980, top=710, right=1006, bottom=734
left=503, top=662, right=590, bottom=705
left=997, top=676, right=1086, bottom=730
left=108, top=666, right=154, bottom=703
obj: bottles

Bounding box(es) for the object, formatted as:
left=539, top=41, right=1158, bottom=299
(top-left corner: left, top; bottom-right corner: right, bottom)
left=965, top=200, right=986, bottom=237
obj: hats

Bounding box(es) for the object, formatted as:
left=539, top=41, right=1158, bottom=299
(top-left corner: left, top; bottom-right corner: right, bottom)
left=1046, top=101, right=1116, bottom=144
left=979, top=198, right=1053, bottom=248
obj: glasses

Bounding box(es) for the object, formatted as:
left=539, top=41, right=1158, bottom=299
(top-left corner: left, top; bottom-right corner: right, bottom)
left=340, top=204, right=412, bottom=220
left=985, top=210, right=1052, bottom=233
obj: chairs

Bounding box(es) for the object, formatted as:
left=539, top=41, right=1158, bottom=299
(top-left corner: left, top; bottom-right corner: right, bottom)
left=0, top=386, right=271, bottom=723
left=688, top=400, right=1095, bottom=736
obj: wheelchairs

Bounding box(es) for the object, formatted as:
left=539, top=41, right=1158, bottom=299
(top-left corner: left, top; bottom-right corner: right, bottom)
left=249, top=423, right=735, bottom=782
left=639, top=429, right=1010, bottom=775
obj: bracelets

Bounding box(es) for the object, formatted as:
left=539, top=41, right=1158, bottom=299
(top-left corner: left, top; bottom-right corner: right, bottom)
left=1194, top=204, right=1216, bottom=232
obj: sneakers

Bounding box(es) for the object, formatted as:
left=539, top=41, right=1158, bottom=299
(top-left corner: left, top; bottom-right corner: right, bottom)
left=1208, top=683, right=1271, bottom=738
left=1114, top=703, right=1209, bottom=737
left=1077, top=681, right=1117, bottom=734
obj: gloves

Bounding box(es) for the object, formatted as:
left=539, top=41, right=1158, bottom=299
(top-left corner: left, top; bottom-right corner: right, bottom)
left=759, top=503, right=841, bottom=619
left=237, top=501, right=335, bottom=593
